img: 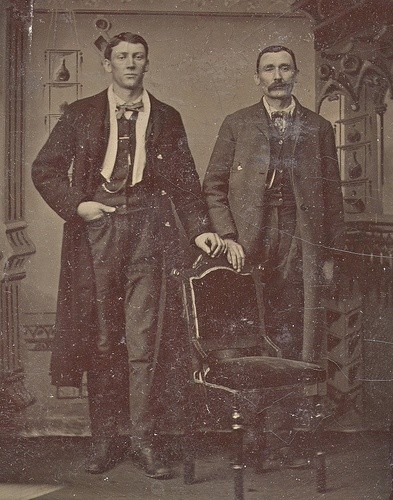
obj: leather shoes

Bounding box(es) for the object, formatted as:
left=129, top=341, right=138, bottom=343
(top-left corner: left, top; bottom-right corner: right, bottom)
left=132, top=447, right=176, bottom=479
left=83, top=438, right=114, bottom=473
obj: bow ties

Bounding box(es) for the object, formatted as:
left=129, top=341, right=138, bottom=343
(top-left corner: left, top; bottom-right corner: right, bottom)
left=117, top=102, right=145, bottom=119
left=271, top=111, right=294, bottom=124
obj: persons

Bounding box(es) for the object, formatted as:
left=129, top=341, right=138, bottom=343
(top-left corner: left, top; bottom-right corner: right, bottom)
left=31, top=28, right=227, bottom=478
left=202, top=46, right=344, bottom=466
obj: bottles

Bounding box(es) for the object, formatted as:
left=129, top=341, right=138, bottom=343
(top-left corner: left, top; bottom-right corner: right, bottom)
left=55, top=59, right=70, bottom=82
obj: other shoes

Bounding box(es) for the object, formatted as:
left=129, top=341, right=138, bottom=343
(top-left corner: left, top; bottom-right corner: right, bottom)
left=272, top=449, right=308, bottom=467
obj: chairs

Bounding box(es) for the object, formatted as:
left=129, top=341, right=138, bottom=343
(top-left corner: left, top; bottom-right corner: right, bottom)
left=170, top=249, right=330, bottom=500
